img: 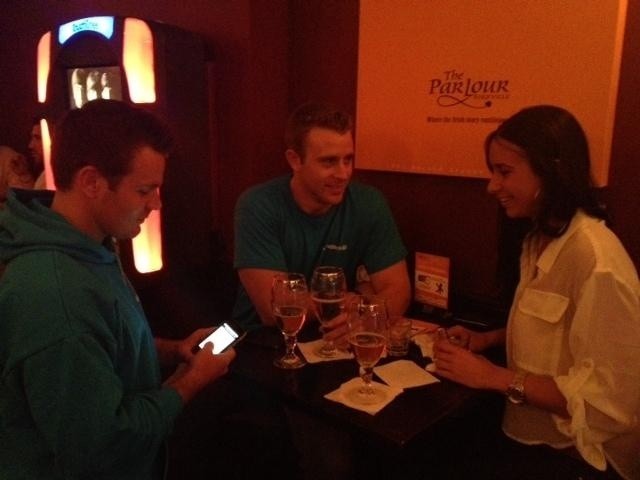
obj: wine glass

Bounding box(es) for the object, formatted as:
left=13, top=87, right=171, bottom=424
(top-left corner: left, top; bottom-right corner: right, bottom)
left=272, top=266, right=387, bottom=404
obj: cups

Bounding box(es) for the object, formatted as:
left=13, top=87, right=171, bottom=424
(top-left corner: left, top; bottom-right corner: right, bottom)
left=387, top=316, right=412, bottom=356
left=435, top=326, right=470, bottom=351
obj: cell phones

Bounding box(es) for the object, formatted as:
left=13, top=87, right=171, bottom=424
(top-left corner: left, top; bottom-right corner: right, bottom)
left=192, top=319, right=247, bottom=357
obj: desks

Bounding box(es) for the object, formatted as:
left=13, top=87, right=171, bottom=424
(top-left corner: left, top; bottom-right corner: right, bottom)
left=224, top=308, right=506, bottom=480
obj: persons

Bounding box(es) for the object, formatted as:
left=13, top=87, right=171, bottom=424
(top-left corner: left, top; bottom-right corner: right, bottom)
left=0, top=98, right=237, bottom=480
left=226, top=103, right=413, bottom=480
left=432, top=103, right=640, bottom=480
left=27, top=112, right=56, bottom=189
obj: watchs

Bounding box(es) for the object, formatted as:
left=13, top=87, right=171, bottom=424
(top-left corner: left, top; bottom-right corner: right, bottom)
left=504, top=370, right=529, bottom=408
